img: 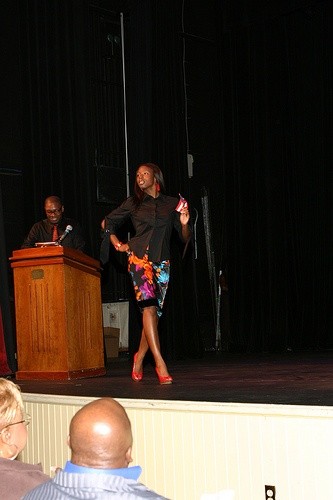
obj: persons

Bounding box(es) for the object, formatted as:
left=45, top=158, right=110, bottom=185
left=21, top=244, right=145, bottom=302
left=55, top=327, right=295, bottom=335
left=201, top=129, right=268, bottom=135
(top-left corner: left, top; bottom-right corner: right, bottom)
left=20, top=196, right=86, bottom=254
left=21, top=396, right=170, bottom=500
left=0, top=378, right=54, bottom=500
left=101, top=162, right=190, bottom=385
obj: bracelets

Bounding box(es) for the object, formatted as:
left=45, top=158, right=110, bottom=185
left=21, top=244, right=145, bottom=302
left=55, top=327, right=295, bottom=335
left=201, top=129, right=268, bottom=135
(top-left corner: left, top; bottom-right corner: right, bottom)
left=115, top=241, right=123, bottom=251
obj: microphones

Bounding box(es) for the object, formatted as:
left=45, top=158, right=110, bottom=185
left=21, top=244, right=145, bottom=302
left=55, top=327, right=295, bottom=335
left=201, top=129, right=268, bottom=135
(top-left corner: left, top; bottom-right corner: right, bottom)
left=56, top=225, right=72, bottom=246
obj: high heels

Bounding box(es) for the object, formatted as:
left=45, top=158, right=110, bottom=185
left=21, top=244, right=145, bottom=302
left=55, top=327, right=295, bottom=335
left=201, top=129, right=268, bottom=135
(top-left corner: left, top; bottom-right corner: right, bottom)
left=156, top=366, right=172, bottom=384
left=132, top=352, right=143, bottom=380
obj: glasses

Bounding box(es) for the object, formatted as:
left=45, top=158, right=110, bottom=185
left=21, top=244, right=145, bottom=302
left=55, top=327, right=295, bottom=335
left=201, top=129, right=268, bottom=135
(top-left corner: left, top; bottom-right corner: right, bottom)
left=46, top=208, right=62, bottom=215
left=0, top=416, right=30, bottom=430
